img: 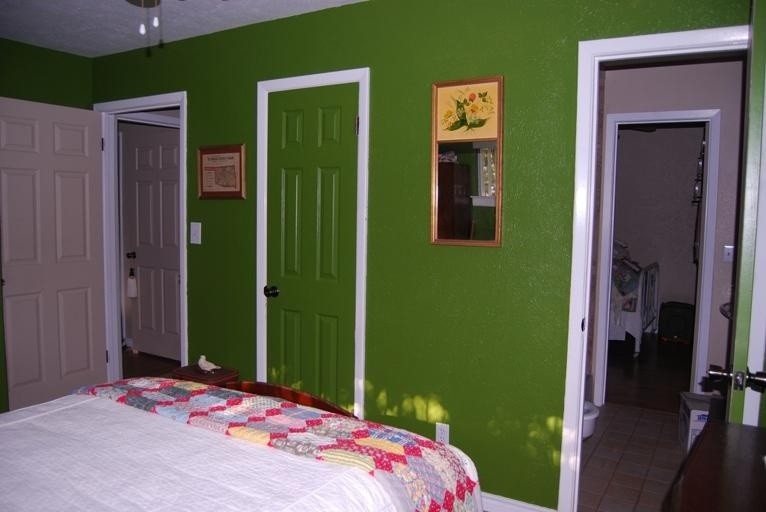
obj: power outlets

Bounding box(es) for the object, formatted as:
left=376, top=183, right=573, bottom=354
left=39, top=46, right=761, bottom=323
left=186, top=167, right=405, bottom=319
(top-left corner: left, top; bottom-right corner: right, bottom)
left=435, top=423, right=449, bottom=444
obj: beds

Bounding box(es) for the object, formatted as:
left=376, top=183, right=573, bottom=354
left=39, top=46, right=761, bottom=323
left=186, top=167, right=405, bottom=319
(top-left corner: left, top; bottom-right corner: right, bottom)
left=0, top=375, right=484, bottom=512
left=608, top=240, right=659, bottom=358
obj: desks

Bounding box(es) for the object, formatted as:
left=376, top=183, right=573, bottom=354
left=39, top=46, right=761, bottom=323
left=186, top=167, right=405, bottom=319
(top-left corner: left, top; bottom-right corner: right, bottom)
left=659, top=418, right=766, bottom=512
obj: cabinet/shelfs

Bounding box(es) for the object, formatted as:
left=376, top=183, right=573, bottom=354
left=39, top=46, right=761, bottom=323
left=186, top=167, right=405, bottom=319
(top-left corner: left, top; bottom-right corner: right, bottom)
left=437, top=163, right=470, bottom=240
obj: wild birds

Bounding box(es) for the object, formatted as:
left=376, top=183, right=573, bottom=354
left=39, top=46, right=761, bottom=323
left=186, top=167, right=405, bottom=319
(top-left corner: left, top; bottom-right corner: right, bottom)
left=197, top=355, right=222, bottom=375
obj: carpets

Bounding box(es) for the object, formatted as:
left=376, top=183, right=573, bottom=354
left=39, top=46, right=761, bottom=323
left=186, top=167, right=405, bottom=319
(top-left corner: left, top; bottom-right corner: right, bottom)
left=605, top=359, right=690, bottom=414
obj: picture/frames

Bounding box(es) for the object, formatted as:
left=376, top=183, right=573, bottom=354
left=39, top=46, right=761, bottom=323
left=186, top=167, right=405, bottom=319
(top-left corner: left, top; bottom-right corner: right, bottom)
left=432, top=75, right=504, bottom=142
left=197, top=144, right=247, bottom=200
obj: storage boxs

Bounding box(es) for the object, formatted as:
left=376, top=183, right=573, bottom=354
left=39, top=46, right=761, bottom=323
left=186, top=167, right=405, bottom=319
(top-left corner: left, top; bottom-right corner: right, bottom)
left=679, top=392, right=709, bottom=454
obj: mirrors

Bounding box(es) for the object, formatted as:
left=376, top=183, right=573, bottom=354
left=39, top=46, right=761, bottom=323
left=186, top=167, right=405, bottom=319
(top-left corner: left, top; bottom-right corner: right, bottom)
left=431, top=140, right=503, bottom=248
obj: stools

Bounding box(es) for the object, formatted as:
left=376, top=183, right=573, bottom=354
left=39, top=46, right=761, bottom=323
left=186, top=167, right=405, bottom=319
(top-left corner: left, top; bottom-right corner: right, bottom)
left=173, top=365, right=240, bottom=387
